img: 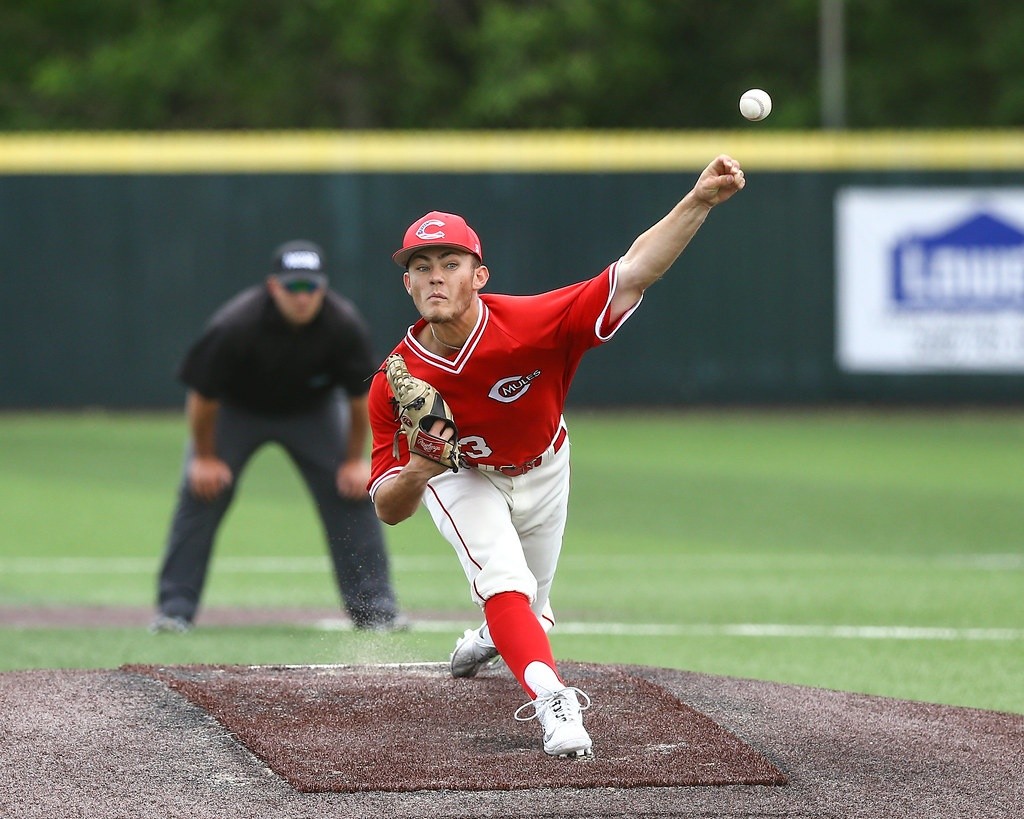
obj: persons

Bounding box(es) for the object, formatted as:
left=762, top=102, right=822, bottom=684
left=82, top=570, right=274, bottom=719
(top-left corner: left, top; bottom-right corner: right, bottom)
left=152, top=239, right=412, bottom=648
left=367, top=153, right=746, bottom=757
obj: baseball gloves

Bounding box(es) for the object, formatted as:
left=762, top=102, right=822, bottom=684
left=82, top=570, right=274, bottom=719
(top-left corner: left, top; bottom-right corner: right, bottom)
left=386, top=353, right=460, bottom=473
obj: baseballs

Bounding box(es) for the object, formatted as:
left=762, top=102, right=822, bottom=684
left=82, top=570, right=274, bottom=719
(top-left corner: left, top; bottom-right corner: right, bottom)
left=740, top=88, right=773, bottom=122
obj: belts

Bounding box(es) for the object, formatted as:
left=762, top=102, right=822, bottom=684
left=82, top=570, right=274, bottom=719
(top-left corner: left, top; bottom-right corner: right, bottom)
left=464, top=427, right=566, bottom=477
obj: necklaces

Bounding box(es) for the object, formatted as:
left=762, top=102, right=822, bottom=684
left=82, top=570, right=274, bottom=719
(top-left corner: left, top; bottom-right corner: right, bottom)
left=429, top=322, right=463, bottom=352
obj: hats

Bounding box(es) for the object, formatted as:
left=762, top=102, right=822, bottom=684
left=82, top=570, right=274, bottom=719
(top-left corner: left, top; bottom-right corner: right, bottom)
left=267, top=239, right=328, bottom=283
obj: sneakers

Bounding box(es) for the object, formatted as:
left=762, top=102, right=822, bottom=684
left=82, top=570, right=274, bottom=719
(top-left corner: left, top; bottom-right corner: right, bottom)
left=451, top=619, right=500, bottom=678
left=536, top=688, right=593, bottom=759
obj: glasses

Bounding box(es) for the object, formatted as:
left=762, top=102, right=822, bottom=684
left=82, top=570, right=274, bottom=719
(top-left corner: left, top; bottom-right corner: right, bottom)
left=285, top=278, right=319, bottom=294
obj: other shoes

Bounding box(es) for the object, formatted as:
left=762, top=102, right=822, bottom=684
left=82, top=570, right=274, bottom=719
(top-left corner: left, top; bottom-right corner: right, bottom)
left=148, top=615, right=191, bottom=637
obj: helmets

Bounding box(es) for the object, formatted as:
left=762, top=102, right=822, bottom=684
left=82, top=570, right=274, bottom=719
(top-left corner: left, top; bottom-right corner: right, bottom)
left=393, top=211, right=482, bottom=269
